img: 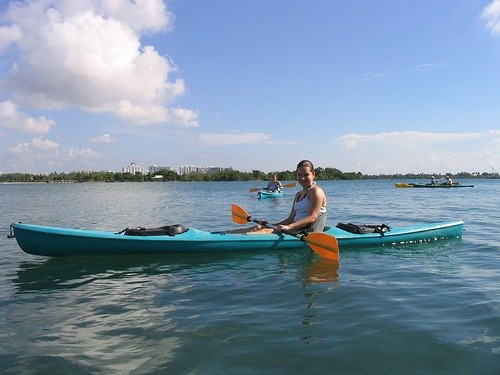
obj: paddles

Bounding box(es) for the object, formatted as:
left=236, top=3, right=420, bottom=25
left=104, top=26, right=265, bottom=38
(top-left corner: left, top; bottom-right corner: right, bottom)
left=249, top=181, right=297, bottom=192
left=231, top=204, right=338, bottom=261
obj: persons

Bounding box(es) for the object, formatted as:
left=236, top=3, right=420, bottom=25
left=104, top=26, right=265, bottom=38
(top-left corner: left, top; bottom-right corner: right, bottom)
left=431, top=176, right=439, bottom=184
left=268, top=175, right=282, bottom=193
left=444, top=176, right=452, bottom=185
left=222, top=160, right=327, bottom=235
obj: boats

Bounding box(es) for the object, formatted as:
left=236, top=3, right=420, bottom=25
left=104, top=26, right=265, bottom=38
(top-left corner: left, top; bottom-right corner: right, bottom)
left=7, top=217, right=460, bottom=259
left=394, top=182, right=474, bottom=188
left=257, top=189, right=286, bottom=201
